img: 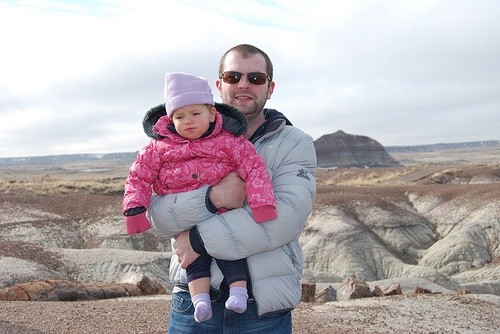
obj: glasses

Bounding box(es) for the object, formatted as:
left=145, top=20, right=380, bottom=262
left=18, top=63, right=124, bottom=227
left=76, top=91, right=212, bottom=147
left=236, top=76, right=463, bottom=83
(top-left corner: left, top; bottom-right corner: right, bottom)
left=220, top=71, right=271, bottom=85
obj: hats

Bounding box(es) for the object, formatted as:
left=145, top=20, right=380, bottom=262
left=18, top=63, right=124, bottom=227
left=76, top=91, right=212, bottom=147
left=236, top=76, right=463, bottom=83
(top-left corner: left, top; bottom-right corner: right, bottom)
left=165, top=73, right=215, bottom=118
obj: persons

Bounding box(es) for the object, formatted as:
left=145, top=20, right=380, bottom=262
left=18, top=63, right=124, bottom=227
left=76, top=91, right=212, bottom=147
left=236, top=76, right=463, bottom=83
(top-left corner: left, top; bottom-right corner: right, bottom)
left=123, top=72, right=277, bottom=323
left=145, top=44, right=317, bottom=334
